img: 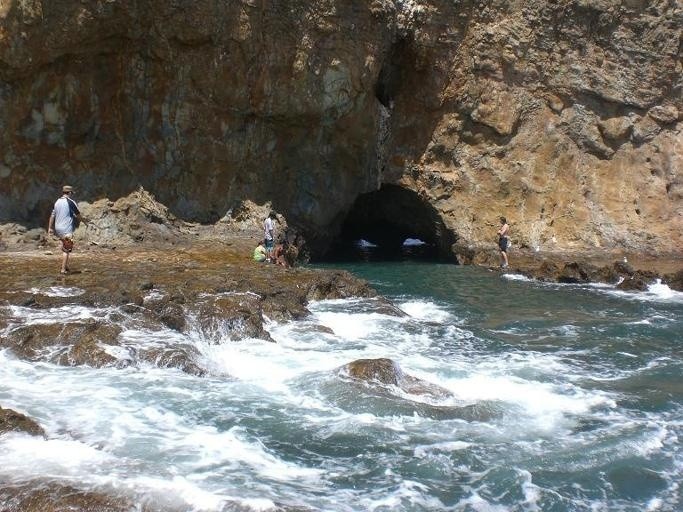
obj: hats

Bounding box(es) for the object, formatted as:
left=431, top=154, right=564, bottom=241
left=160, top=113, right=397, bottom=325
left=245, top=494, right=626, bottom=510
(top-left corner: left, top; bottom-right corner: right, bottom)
left=61, top=185, right=75, bottom=194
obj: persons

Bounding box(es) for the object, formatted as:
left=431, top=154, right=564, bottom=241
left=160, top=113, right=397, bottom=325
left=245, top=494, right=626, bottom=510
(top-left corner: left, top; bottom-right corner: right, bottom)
left=263, top=211, right=276, bottom=263
left=496, top=216, right=509, bottom=268
left=47, top=184, right=81, bottom=275
left=253, top=240, right=268, bottom=263
